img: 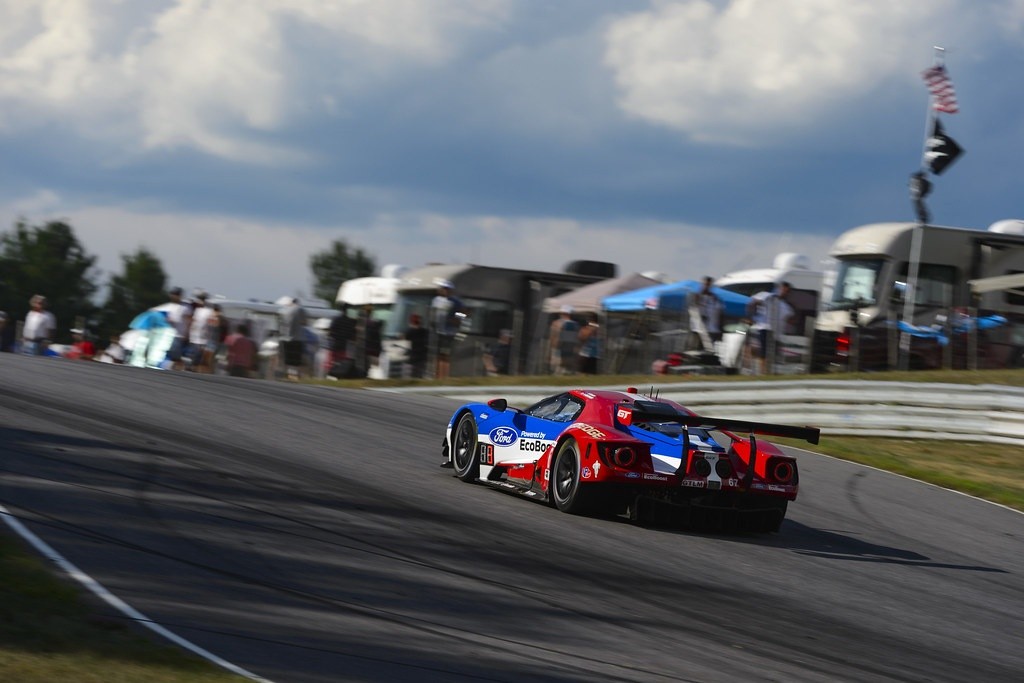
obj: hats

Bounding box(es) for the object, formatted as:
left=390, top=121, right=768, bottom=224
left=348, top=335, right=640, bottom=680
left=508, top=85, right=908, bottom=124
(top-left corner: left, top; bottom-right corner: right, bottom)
left=29, top=295, right=51, bottom=308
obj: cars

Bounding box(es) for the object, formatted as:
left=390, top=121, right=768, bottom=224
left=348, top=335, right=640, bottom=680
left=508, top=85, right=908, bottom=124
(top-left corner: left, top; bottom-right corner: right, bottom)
left=441, top=386, right=799, bottom=531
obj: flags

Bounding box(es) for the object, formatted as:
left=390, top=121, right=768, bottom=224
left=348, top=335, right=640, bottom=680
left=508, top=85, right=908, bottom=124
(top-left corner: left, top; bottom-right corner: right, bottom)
left=908, top=171, right=934, bottom=225
left=921, top=116, right=967, bottom=175
left=920, top=65, right=960, bottom=113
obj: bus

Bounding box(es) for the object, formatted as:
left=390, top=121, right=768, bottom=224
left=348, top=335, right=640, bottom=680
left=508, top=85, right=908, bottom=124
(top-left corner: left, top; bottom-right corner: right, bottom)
left=715, top=251, right=823, bottom=348
left=333, top=259, right=621, bottom=370
left=817, top=219, right=1024, bottom=363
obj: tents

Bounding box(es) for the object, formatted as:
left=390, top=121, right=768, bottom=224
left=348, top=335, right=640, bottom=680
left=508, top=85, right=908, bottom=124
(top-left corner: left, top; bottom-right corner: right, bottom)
left=541, top=271, right=766, bottom=376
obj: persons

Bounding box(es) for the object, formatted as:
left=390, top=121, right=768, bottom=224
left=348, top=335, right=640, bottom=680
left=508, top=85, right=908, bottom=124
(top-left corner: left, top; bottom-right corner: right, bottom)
left=20, top=276, right=797, bottom=382
